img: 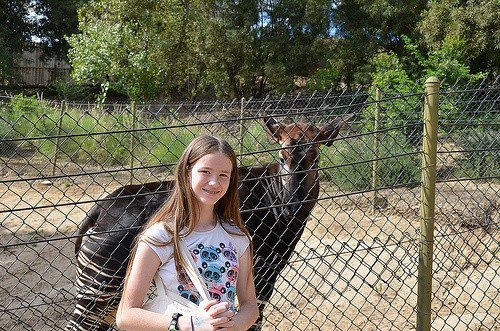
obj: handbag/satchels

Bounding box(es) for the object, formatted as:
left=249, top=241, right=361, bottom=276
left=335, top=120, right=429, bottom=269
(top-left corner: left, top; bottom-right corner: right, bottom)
left=140, top=231, right=215, bottom=319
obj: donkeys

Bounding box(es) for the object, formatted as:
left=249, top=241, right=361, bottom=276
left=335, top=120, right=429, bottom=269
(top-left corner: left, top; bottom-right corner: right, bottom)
left=64, top=112, right=357, bottom=331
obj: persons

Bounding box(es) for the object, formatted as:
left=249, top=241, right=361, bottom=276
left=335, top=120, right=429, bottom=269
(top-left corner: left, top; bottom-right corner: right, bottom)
left=114, top=135, right=260, bottom=331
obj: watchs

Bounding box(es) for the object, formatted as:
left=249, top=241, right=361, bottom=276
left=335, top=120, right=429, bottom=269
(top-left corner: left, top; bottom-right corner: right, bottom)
left=167, top=313, right=183, bottom=331
